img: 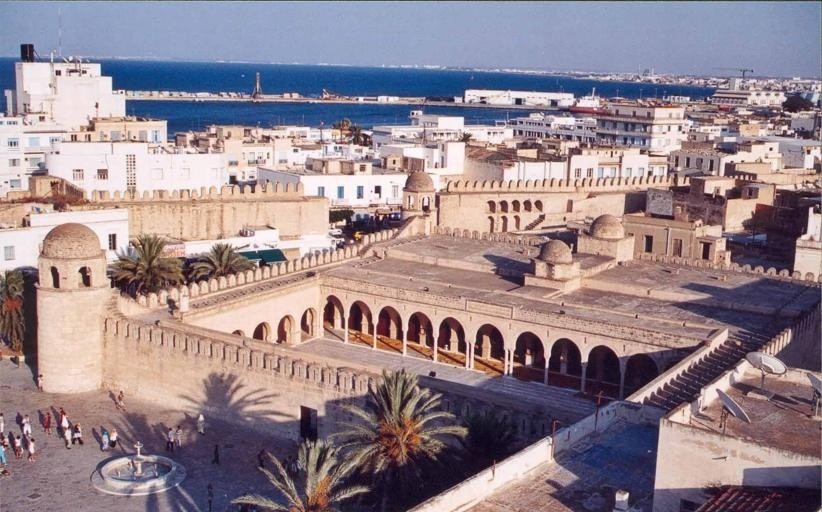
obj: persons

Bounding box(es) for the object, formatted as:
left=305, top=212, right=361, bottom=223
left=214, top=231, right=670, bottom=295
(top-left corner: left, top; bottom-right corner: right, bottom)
left=43, top=412, right=51, bottom=434
left=211, top=444, right=219, bottom=464
left=109, top=427, right=117, bottom=448
left=38, top=374, right=44, bottom=392
left=258, top=448, right=266, bottom=467
left=73, top=423, right=84, bottom=445
left=102, top=429, right=109, bottom=452
left=116, top=391, right=127, bottom=411
left=58, top=407, right=67, bottom=428
left=64, top=426, right=73, bottom=449
left=0, top=413, right=6, bottom=434
left=0, top=415, right=37, bottom=468
left=175, top=425, right=183, bottom=449
left=165, top=427, right=175, bottom=453
left=280, top=454, right=302, bottom=481
left=197, top=413, right=206, bottom=435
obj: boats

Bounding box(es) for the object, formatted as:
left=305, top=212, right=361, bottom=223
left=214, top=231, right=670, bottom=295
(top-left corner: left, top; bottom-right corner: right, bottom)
left=570, top=88, right=608, bottom=115
